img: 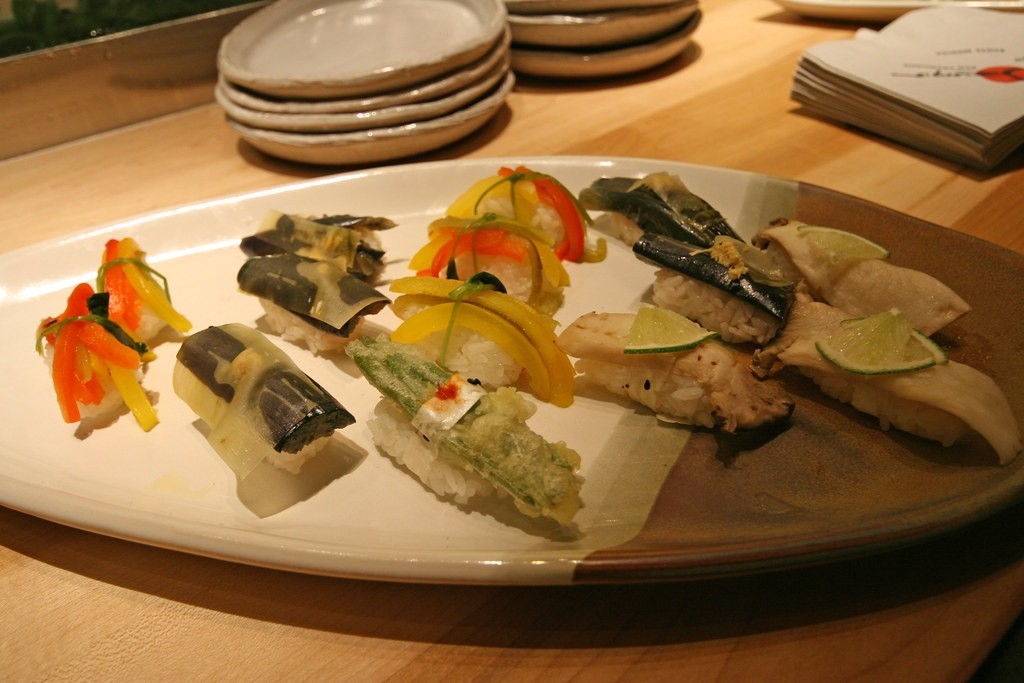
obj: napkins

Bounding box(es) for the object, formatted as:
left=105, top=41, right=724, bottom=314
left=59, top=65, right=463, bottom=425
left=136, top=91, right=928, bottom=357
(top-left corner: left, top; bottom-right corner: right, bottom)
left=788, top=6, right=1024, bottom=175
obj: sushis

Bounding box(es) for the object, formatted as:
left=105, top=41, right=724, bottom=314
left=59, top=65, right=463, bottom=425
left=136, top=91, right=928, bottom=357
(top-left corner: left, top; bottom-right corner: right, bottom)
left=33, top=165, right=1022, bottom=529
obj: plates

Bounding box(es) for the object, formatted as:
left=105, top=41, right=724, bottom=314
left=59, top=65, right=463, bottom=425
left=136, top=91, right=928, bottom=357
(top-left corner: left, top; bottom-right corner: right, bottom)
left=213, top=0, right=702, bottom=168
left=771, top=0, right=1023, bottom=25
left=2, top=154, right=1024, bottom=586
